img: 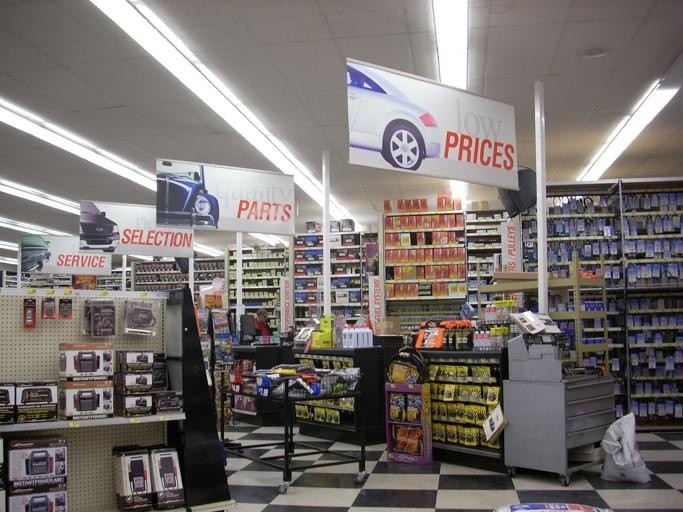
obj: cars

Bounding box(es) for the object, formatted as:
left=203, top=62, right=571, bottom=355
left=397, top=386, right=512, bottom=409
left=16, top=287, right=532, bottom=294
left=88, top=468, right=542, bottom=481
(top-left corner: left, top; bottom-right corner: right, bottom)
left=22, top=237, right=55, bottom=278
left=79, top=201, right=120, bottom=254
left=345, top=58, right=446, bottom=173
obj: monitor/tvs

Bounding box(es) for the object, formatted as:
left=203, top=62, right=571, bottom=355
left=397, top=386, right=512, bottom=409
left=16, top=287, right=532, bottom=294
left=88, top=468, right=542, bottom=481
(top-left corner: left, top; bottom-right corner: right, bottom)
left=240, top=315, right=256, bottom=336
left=495, top=169, right=537, bottom=219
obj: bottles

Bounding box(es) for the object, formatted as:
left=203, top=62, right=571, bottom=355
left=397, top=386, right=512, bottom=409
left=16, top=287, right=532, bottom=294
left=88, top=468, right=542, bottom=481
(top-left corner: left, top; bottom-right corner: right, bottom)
left=473, top=299, right=519, bottom=351
left=341, top=323, right=374, bottom=349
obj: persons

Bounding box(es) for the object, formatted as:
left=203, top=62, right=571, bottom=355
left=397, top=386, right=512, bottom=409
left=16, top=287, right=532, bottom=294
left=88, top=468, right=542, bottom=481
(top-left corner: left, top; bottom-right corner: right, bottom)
left=251, top=307, right=271, bottom=339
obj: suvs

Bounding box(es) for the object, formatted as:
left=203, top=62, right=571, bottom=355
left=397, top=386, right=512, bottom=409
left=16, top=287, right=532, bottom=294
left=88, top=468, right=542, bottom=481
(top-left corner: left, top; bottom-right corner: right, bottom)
left=156, top=159, right=222, bottom=231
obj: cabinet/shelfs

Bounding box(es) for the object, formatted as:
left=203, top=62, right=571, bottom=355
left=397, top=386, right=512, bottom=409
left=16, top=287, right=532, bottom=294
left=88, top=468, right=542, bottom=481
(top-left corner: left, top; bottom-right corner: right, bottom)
left=0, top=287, right=238, bottom=511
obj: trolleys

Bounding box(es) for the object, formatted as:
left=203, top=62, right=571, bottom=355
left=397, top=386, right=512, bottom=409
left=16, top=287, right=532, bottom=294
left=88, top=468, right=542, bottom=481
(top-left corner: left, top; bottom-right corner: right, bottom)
left=214, top=366, right=370, bottom=495
